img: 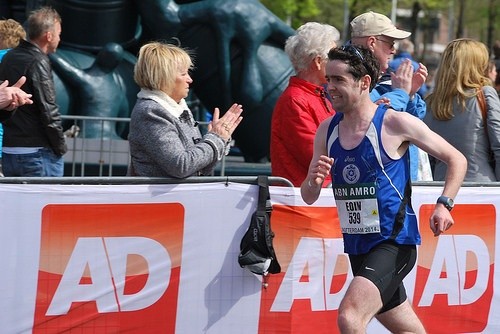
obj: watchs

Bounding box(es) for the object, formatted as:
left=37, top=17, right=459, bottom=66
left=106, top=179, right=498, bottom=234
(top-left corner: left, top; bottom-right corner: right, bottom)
left=437, top=196, right=455, bottom=211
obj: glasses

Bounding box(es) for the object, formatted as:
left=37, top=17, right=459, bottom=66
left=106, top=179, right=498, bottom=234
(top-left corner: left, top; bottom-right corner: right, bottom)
left=376, top=38, right=395, bottom=50
left=338, top=44, right=363, bottom=59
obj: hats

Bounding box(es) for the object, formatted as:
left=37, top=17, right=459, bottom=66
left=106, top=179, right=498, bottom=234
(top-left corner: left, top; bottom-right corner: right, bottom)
left=350, top=11, right=412, bottom=39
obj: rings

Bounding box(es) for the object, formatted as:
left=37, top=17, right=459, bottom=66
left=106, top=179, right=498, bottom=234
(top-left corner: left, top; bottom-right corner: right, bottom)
left=226, top=128, right=230, bottom=131
left=222, top=123, right=227, bottom=128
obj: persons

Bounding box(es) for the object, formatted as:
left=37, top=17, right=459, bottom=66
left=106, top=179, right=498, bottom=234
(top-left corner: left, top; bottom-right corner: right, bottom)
left=1, top=6, right=68, bottom=179
left=126, top=42, right=245, bottom=178
left=300, top=43, right=467, bottom=334
left=269, top=9, right=500, bottom=183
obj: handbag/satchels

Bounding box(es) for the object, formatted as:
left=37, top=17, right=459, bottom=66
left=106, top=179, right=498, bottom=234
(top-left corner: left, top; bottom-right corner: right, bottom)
left=238, top=175, right=282, bottom=276
